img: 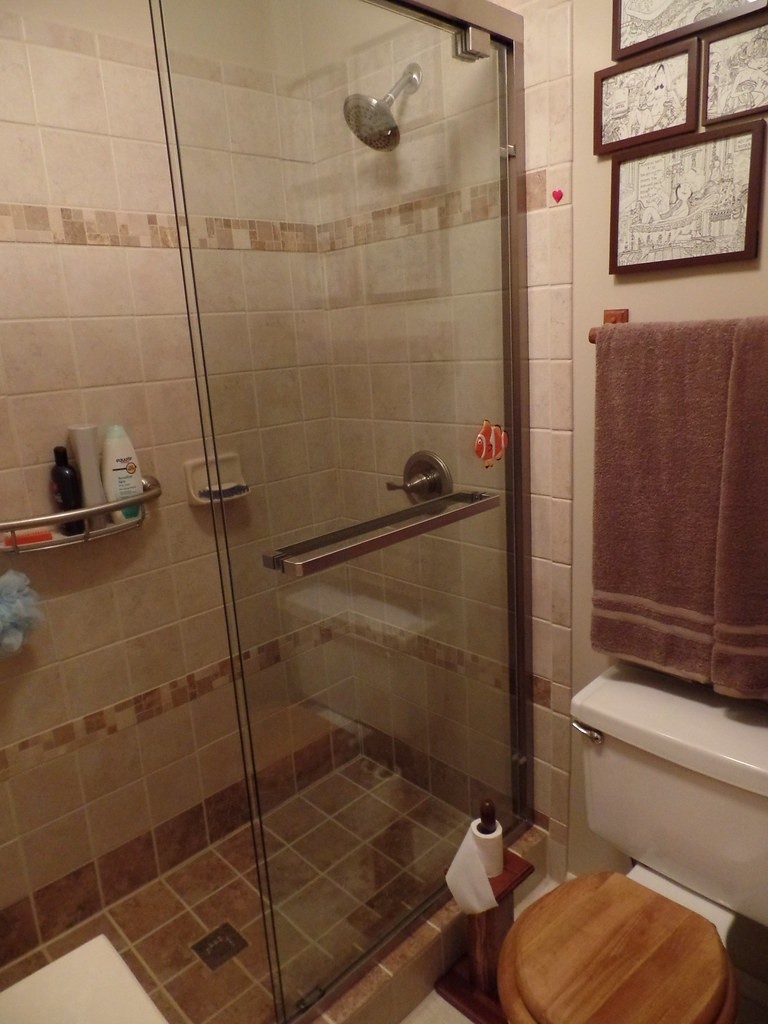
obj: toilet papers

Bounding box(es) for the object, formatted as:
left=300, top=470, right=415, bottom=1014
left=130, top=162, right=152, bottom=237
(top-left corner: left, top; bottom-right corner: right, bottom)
left=445, top=817, right=503, bottom=915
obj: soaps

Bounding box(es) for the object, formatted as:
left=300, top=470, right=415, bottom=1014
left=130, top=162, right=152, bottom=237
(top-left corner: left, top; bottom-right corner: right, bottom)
left=206, top=482, right=239, bottom=491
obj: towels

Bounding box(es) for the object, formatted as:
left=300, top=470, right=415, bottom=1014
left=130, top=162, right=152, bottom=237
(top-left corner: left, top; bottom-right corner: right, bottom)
left=590, top=316, right=768, bottom=699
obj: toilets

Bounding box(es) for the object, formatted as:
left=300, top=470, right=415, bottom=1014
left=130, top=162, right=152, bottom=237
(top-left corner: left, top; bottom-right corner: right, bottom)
left=496, top=662, right=768, bottom=1024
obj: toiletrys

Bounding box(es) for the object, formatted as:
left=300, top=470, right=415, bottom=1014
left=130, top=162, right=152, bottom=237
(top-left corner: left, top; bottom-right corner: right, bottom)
left=67, top=423, right=107, bottom=532
left=51, top=446, right=81, bottom=536
left=101, top=425, right=144, bottom=524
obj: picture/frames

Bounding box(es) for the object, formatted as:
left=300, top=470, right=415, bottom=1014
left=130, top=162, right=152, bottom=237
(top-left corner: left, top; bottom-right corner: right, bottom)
left=611, top=0, right=768, bottom=62
left=593, top=37, right=702, bottom=156
left=700, top=7, right=768, bottom=126
left=608, top=118, right=767, bottom=275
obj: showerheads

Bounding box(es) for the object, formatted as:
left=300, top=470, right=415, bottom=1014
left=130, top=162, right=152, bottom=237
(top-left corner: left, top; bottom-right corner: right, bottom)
left=343, top=62, right=422, bottom=153
